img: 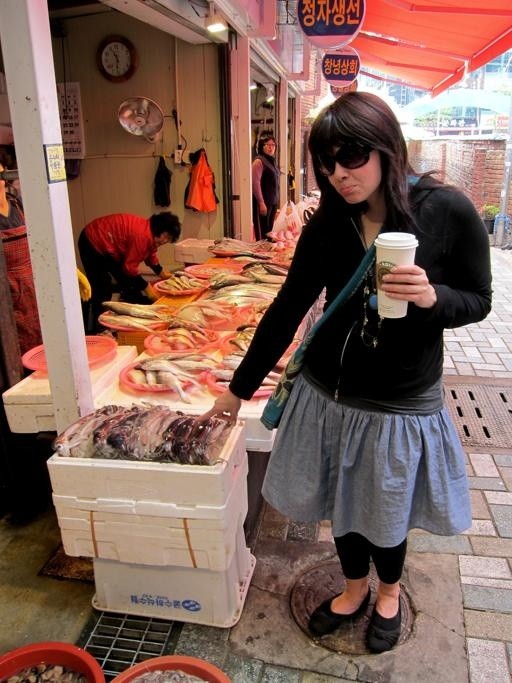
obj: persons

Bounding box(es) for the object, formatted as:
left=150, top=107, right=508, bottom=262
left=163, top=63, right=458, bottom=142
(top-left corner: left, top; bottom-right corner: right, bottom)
left=252, top=135, right=281, bottom=243
left=0, top=163, right=92, bottom=375
left=194, top=90, right=494, bottom=655
left=78, top=210, right=181, bottom=334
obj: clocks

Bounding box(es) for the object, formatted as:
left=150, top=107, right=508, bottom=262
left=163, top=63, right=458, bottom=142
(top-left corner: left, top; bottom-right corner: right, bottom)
left=94, top=33, right=139, bottom=83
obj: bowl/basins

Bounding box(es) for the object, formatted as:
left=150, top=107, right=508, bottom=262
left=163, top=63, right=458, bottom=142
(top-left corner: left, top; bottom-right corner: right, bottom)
left=0, top=642, right=232, bottom=683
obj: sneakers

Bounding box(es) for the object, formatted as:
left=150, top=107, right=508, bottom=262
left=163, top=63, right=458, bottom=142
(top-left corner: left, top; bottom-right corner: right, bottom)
left=368, top=595, right=401, bottom=653
left=308, top=585, right=371, bottom=636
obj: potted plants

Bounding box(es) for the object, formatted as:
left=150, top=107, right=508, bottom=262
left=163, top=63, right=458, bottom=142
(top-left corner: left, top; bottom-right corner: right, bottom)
left=480, top=203, right=500, bottom=233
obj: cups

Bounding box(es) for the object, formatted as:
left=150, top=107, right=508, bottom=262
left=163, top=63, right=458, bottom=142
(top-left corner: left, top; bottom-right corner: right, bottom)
left=373, top=232, right=418, bottom=320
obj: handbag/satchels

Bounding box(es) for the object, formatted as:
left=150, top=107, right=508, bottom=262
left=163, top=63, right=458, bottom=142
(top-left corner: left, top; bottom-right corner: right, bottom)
left=261, top=354, right=303, bottom=429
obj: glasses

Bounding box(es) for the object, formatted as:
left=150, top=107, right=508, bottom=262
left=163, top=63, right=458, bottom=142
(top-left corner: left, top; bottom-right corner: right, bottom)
left=316, top=147, right=376, bottom=176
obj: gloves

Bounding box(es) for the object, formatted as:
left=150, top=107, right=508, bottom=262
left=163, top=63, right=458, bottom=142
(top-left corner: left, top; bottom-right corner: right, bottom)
left=145, top=282, right=160, bottom=300
left=160, top=269, right=172, bottom=279
left=77, top=269, right=92, bottom=301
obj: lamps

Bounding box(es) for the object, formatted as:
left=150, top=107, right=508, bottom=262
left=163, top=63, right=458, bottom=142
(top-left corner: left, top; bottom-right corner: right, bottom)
left=204, top=3, right=227, bottom=34
